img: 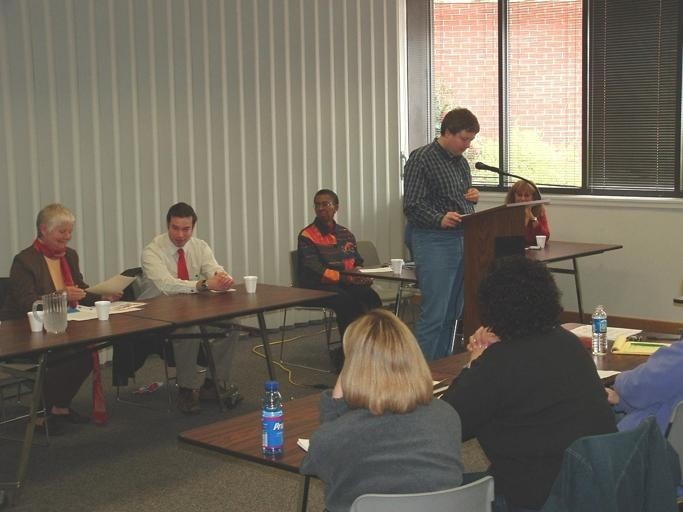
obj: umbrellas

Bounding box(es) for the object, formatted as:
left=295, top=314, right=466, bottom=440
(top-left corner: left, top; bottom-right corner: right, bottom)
left=198, top=383, right=243, bottom=402
left=48, top=407, right=90, bottom=424
left=34, top=414, right=64, bottom=437
left=174, top=389, right=194, bottom=414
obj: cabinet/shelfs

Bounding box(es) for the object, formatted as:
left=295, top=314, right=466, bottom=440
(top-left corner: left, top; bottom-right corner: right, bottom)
left=590, top=304, right=609, bottom=357
left=259, top=380, right=285, bottom=457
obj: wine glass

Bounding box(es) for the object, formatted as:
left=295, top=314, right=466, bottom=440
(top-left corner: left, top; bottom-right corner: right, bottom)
left=472, top=337, right=477, bottom=343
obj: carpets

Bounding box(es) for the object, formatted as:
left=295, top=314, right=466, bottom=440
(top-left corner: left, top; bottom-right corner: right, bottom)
left=176, top=248, right=189, bottom=281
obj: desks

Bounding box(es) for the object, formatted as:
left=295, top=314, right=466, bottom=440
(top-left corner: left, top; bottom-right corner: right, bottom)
left=0, top=242, right=682, bottom=510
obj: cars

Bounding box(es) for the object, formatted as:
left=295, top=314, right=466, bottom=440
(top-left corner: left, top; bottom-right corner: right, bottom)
left=93, top=300, right=111, bottom=321
left=535, top=236, right=546, bottom=249
left=390, top=258, right=403, bottom=275
left=242, top=275, right=258, bottom=293
left=26, top=310, right=44, bottom=333
left=31, top=292, right=70, bottom=335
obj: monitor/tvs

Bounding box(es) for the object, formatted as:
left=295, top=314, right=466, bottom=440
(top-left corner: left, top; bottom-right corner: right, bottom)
left=609, top=334, right=671, bottom=357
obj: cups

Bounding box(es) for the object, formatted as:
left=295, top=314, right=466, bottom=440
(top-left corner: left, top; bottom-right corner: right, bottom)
left=312, top=201, right=334, bottom=210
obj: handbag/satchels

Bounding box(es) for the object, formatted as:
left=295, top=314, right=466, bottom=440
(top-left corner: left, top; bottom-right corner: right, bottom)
left=530, top=216, right=537, bottom=222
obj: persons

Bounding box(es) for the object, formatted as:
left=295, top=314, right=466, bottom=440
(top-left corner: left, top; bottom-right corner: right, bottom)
left=603, top=338, right=682, bottom=499
left=8, top=203, right=123, bottom=435
left=505, top=179, right=550, bottom=250
left=295, top=189, right=383, bottom=374
left=401, top=108, right=480, bottom=364
left=439, top=255, right=618, bottom=512
left=136, top=202, right=235, bottom=414
left=298, top=306, right=464, bottom=512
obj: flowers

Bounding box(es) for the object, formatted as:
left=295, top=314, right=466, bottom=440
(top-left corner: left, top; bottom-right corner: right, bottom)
left=475, top=162, right=541, bottom=200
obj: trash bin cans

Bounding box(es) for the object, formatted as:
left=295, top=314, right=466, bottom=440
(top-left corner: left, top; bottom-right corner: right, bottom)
left=201, top=279, right=208, bottom=290
